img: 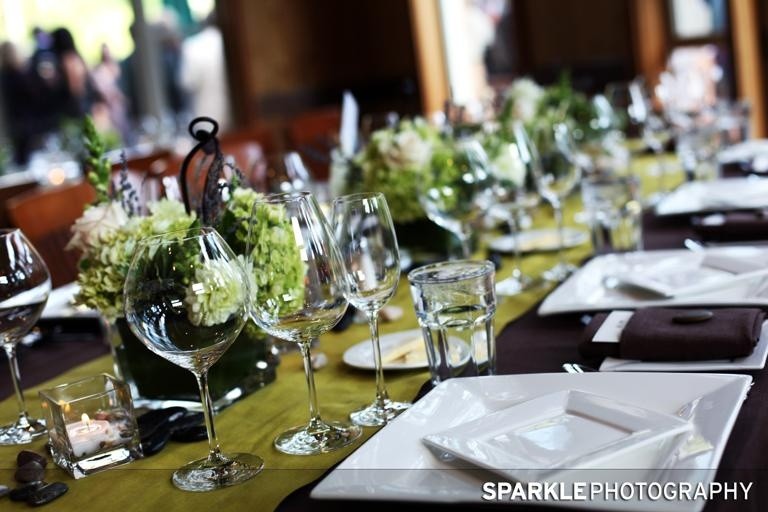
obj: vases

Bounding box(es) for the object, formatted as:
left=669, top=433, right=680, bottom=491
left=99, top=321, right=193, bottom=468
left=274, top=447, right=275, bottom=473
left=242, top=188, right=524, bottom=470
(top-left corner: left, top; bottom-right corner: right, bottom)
left=115, top=308, right=279, bottom=415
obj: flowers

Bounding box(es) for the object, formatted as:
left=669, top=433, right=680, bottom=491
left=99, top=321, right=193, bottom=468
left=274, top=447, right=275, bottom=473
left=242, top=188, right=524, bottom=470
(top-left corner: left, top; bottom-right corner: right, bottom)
left=61, top=109, right=308, bottom=340
left=350, top=116, right=460, bottom=221
left=482, top=73, right=598, bottom=161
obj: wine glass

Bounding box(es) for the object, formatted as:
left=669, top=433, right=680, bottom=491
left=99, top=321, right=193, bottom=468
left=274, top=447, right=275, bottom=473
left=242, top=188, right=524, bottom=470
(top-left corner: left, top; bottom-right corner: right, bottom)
left=242, top=184, right=366, bottom=460
left=118, top=221, right=267, bottom=494
left=408, top=67, right=721, bottom=296
left=0, top=221, right=54, bottom=446
left=326, top=189, right=418, bottom=433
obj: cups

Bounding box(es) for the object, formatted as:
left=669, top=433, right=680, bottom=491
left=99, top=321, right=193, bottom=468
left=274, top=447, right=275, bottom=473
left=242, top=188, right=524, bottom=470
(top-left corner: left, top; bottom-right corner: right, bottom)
left=406, top=255, right=510, bottom=387
left=34, top=369, right=147, bottom=483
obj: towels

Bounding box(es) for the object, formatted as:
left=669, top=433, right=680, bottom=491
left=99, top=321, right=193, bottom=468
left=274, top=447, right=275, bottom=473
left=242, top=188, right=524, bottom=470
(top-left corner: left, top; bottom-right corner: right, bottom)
left=578, top=307, right=767, bottom=361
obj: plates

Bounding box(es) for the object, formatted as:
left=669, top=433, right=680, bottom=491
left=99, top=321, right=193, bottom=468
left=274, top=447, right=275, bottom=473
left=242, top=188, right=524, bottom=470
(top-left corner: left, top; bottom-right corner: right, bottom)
left=598, top=316, right=768, bottom=376
left=306, top=371, right=754, bottom=512
left=419, top=385, right=700, bottom=493
left=340, top=327, right=474, bottom=374
left=534, top=131, right=768, bottom=322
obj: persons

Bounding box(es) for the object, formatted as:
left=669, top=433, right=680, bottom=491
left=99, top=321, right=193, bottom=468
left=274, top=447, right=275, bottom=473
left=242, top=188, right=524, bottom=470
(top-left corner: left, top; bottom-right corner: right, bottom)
left=0, top=9, right=232, bottom=178
